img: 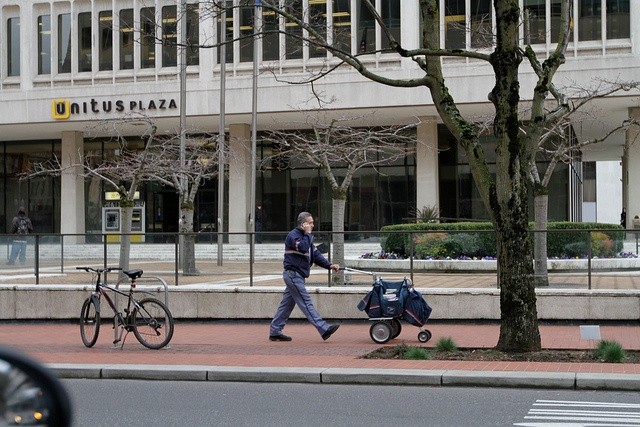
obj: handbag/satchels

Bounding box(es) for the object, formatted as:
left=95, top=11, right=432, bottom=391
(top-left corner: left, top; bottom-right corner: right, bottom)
left=357, top=284, right=380, bottom=317
left=403, top=290, right=432, bottom=327
left=380, top=279, right=410, bottom=315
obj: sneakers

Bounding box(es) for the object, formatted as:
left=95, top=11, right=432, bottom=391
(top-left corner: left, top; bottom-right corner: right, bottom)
left=270, top=334, right=291, bottom=341
left=322, top=324, right=339, bottom=340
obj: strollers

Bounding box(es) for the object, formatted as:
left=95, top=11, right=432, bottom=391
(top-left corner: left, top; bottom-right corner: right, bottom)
left=337, top=267, right=431, bottom=343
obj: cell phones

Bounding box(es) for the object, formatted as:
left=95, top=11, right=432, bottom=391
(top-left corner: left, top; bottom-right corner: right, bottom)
left=302, top=222, right=309, bottom=228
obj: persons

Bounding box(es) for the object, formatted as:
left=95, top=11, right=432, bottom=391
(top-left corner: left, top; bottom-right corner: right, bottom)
left=621, top=207, right=626, bottom=229
left=6, top=206, right=33, bottom=265
left=269, top=211, right=340, bottom=340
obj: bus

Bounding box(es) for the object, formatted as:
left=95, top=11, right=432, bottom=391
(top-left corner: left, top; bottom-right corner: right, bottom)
left=5, top=385, right=49, bottom=425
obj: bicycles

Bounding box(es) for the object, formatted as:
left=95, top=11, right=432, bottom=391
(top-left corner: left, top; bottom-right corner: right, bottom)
left=76, top=267, right=174, bottom=349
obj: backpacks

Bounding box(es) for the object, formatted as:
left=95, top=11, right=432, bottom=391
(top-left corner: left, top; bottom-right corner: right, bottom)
left=17, top=216, right=29, bottom=240
